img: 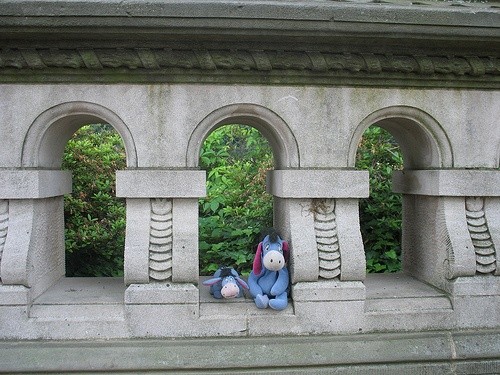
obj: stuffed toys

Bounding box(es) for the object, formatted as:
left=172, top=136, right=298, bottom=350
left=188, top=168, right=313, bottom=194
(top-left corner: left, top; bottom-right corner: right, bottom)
left=247, top=227, right=291, bottom=310
left=203, top=266, right=250, bottom=300
